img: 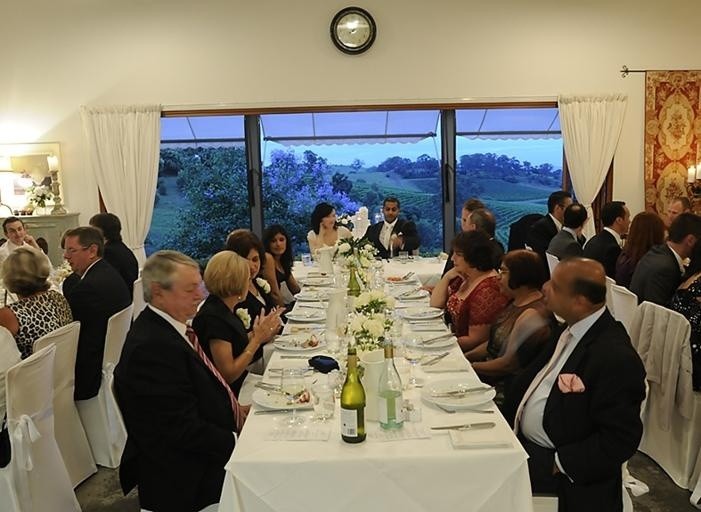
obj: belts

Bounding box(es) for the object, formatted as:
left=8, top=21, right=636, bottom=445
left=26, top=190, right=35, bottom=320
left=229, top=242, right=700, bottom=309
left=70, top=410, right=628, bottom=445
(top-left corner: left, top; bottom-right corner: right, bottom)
left=279, top=365, right=306, bottom=427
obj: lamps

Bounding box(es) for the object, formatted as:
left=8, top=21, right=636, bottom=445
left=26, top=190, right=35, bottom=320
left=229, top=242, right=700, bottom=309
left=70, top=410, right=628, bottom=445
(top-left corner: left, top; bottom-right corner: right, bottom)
left=0, top=341, right=87, bottom=512
left=72, top=304, right=129, bottom=472
left=533, top=378, right=650, bottom=512
left=29, top=320, right=96, bottom=490
left=628, top=301, right=701, bottom=494
left=521, top=242, right=639, bottom=337
left=686, top=164, right=700, bottom=197
left=1, top=271, right=143, bottom=332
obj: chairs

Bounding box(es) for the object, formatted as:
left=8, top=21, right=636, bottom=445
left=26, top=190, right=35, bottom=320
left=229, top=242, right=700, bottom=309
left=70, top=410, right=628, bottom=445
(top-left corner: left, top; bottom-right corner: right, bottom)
left=533, top=378, right=650, bottom=512
left=29, top=320, right=96, bottom=490
left=72, top=304, right=129, bottom=472
left=1, top=271, right=143, bottom=332
left=628, top=301, right=701, bottom=494
left=521, top=242, right=639, bottom=337
left=0, top=341, right=87, bottom=512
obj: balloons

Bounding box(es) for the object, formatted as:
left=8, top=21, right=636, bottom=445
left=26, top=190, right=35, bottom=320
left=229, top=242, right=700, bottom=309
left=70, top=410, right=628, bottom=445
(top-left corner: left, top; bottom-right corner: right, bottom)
left=328, top=6, right=377, bottom=55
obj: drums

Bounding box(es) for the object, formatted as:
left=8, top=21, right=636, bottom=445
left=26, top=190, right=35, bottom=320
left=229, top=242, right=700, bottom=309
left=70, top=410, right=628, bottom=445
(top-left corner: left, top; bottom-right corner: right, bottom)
left=432, top=421, right=497, bottom=433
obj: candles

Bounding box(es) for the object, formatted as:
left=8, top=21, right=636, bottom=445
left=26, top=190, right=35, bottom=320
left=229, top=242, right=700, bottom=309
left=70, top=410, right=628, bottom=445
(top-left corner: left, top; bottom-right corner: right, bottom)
left=46, top=156, right=58, bottom=170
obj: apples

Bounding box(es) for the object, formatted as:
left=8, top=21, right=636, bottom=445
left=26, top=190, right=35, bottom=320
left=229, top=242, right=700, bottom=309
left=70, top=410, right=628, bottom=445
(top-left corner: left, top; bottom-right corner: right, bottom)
left=46, top=156, right=58, bottom=170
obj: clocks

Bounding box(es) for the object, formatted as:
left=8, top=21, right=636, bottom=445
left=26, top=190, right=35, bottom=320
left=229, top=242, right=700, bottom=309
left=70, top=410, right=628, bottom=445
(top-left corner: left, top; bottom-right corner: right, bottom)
left=328, top=6, right=377, bottom=55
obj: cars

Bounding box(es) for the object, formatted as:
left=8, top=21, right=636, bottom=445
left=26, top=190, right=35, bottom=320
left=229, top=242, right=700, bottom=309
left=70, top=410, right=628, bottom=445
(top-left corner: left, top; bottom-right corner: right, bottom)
left=366, top=258, right=385, bottom=292
left=398, top=251, right=408, bottom=264
left=362, top=351, right=386, bottom=422
left=301, top=253, right=311, bottom=267
left=311, top=386, right=334, bottom=421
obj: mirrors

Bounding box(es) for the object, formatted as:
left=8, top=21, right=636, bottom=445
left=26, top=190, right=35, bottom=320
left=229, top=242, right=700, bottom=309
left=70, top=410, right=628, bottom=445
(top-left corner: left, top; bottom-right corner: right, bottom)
left=0, top=142, right=65, bottom=207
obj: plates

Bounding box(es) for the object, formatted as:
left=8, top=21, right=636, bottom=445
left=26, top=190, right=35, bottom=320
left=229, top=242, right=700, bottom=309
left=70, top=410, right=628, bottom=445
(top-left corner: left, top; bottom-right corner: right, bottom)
left=0, top=212, right=80, bottom=268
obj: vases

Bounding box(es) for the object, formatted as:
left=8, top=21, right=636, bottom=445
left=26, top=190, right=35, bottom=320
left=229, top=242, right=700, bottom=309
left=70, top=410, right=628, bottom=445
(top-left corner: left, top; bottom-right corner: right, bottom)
left=25, top=184, right=51, bottom=208
left=329, top=212, right=395, bottom=351
left=35, top=201, right=46, bottom=215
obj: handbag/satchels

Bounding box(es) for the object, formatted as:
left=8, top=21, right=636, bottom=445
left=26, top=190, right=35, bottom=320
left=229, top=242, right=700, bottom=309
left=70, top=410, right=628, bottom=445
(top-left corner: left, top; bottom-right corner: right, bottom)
left=243, top=348, right=255, bottom=357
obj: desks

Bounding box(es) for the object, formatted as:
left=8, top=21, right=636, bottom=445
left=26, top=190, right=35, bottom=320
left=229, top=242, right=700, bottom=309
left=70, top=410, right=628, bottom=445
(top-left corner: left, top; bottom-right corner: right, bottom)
left=289, top=255, right=447, bottom=288
left=219, top=260, right=532, bottom=512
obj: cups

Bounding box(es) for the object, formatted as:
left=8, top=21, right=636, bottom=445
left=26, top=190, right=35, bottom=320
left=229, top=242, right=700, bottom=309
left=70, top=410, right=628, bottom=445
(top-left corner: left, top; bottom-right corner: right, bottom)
left=63, top=246, right=89, bottom=255
left=498, top=268, right=510, bottom=274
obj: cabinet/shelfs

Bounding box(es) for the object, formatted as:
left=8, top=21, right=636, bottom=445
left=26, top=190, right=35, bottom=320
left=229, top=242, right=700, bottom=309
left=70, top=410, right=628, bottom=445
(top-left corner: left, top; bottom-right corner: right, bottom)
left=339, top=348, right=367, bottom=444
left=377, top=344, right=403, bottom=433
left=0, top=212, right=80, bottom=268
left=346, top=247, right=362, bottom=296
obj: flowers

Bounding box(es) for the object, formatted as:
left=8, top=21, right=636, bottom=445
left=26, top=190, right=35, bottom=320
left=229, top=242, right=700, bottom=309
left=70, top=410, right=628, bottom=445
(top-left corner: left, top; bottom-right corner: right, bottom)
left=25, top=184, right=51, bottom=208
left=329, top=212, right=395, bottom=351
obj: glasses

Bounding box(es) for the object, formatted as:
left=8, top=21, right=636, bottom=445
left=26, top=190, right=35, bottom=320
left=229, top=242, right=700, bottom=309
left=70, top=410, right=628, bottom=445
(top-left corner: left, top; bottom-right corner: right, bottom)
left=686, top=164, right=700, bottom=197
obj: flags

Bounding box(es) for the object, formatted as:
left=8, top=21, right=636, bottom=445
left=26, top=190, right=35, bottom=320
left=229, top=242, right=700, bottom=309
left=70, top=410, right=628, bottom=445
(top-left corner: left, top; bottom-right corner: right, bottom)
left=35, top=201, right=46, bottom=215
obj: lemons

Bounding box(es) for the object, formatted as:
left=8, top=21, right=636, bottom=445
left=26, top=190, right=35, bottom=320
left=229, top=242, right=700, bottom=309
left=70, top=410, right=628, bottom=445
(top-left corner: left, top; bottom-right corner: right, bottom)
left=313, top=286, right=349, bottom=351
left=313, top=245, right=334, bottom=274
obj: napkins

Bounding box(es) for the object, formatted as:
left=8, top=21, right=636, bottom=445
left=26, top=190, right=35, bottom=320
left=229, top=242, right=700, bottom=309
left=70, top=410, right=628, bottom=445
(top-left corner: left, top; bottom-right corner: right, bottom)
left=449, top=426, right=513, bottom=449
left=424, top=359, right=470, bottom=373
left=268, top=358, right=310, bottom=373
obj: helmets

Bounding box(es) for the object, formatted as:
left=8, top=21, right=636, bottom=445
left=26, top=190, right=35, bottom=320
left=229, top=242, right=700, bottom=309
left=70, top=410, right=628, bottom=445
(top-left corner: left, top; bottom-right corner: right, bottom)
left=384, top=271, right=499, bottom=411
left=250, top=276, right=333, bottom=414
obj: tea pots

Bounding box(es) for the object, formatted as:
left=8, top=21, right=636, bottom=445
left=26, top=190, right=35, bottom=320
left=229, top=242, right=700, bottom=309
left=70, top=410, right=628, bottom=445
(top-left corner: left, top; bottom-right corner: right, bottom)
left=0, top=142, right=65, bottom=207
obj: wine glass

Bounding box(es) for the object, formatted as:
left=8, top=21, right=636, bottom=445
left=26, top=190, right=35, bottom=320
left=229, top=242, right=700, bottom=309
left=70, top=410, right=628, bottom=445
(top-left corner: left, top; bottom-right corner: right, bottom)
left=269, top=327, right=274, bottom=332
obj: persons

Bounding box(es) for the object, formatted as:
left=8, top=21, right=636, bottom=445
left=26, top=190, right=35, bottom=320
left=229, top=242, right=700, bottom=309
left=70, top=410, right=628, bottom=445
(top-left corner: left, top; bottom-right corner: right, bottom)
left=191, top=249, right=285, bottom=402
left=1, top=217, right=56, bottom=279
left=262, top=227, right=303, bottom=310
left=428, top=230, right=505, bottom=354
left=306, top=202, right=356, bottom=261
left=614, top=212, right=665, bottom=288
left=547, top=204, right=588, bottom=259
left=668, top=197, right=691, bottom=224
left=525, top=192, right=586, bottom=250
left=89, top=212, right=141, bottom=283
left=670, top=255, right=701, bottom=393
left=358, top=196, right=423, bottom=258
left=465, top=208, right=505, bottom=270
left=497, top=257, right=647, bottom=512
left=0, top=246, right=75, bottom=361
left=441, top=198, right=484, bottom=278
left=583, top=201, right=631, bottom=279
left=466, top=249, right=557, bottom=416
left=0, top=323, right=25, bottom=469
left=224, top=228, right=288, bottom=319
left=58, top=226, right=135, bottom=404
left=111, top=248, right=252, bottom=512
left=630, top=213, right=700, bottom=305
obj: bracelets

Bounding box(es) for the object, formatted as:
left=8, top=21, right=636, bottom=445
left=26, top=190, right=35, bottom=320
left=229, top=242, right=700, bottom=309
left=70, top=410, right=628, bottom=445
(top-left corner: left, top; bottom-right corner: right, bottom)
left=445, top=274, right=450, bottom=281
left=243, top=348, right=255, bottom=357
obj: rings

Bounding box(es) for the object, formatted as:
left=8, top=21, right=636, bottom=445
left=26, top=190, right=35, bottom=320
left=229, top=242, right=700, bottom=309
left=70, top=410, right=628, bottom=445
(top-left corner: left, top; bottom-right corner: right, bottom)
left=269, top=327, right=274, bottom=332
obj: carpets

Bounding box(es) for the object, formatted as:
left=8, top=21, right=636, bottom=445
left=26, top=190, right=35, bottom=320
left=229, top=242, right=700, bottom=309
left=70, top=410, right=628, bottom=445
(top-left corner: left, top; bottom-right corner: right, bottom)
left=514, top=334, right=573, bottom=435
left=186, top=324, right=243, bottom=440
